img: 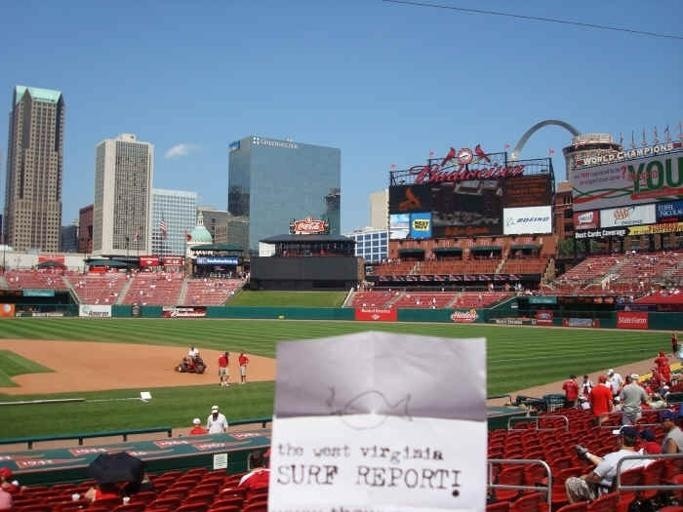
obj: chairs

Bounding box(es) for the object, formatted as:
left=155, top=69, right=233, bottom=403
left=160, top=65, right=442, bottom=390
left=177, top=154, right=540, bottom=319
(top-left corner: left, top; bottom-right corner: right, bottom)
left=1, top=372, right=683, bottom=511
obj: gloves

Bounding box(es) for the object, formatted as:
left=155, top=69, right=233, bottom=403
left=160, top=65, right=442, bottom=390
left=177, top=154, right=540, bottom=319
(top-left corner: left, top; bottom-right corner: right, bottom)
left=575, top=444, right=590, bottom=460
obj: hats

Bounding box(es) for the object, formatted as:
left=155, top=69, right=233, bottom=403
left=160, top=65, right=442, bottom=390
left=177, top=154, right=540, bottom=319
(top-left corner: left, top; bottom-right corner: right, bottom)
left=211, top=405, right=218, bottom=414
left=599, top=369, right=675, bottom=441
left=250, top=451, right=263, bottom=465
left=192, top=418, right=201, bottom=424
left=1, top=467, right=11, bottom=478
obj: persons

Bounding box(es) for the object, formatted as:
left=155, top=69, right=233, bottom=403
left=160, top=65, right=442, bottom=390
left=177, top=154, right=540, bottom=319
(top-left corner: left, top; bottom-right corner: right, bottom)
left=238, top=352, right=248, bottom=384
left=357, top=283, right=400, bottom=296
left=381, top=256, right=400, bottom=266
left=205, top=404, right=228, bottom=434
left=0, top=487, right=15, bottom=511
left=669, top=332, right=678, bottom=357
left=181, top=346, right=199, bottom=368
left=236, top=451, right=271, bottom=492
left=0, top=466, right=20, bottom=493
left=558, top=351, right=682, bottom=504
left=584, top=250, right=680, bottom=312
left=216, top=351, right=230, bottom=386
left=190, top=416, right=206, bottom=434
left=0, top=265, right=249, bottom=310
left=82, top=474, right=153, bottom=501
left=487, top=281, right=533, bottom=295
left=432, top=283, right=445, bottom=309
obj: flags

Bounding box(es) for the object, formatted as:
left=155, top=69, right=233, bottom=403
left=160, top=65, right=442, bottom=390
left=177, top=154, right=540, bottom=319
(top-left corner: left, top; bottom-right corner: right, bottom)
left=159, top=220, right=167, bottom=239
left=133, top=230, right=140, bottom=241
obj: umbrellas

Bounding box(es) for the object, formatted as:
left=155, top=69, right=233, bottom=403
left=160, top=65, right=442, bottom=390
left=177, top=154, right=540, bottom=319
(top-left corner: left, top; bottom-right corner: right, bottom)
left=71, top=450, right=147, bottom=483
